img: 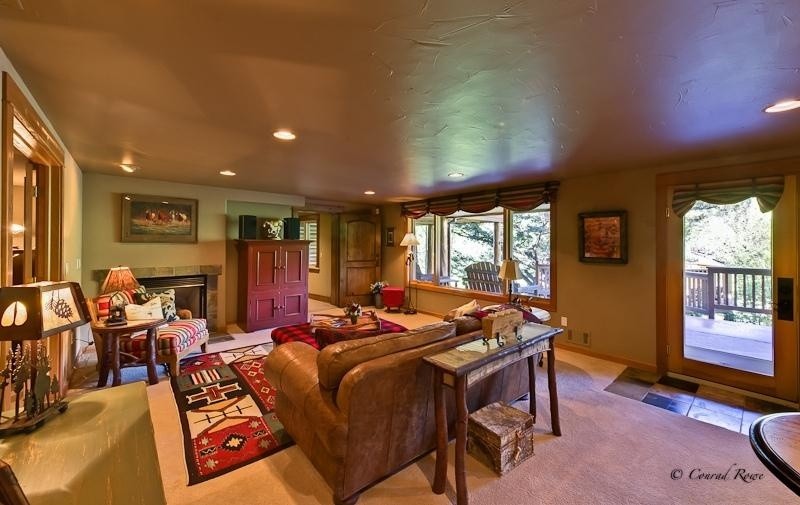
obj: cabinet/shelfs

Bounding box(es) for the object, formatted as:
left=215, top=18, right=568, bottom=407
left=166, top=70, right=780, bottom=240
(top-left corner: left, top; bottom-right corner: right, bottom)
left=235, top=238, right=313, bottom=332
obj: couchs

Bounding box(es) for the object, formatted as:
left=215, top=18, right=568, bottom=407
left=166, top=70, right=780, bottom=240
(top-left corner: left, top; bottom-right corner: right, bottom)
left=264, top=305, right=552, bottom=505
left=83, top=286, right=210, bottom=376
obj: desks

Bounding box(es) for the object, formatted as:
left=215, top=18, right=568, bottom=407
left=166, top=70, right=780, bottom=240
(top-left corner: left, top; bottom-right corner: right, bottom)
left=424, top=323, right=563, bottom=505
left=1, top=382, right=166, bottom=505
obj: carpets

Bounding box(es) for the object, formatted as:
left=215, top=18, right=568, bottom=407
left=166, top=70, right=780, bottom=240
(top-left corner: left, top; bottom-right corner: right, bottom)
left=166, top=341, right=295, bottom=486
left=145, top=305, right=800, bottom=505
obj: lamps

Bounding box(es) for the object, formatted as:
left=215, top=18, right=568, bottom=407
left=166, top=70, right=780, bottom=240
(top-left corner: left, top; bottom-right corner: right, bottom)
left=498, top=258, right=522, bottom=305
left=0, top=281, right=91, bottom=431
left=102, top=265, right=140, bottom=325
left=400, top=233, right=419, bottom=314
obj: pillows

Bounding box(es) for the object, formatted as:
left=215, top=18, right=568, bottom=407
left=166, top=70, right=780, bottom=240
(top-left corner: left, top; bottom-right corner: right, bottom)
left=135, top=289, right=181, bottom=322
left=445, top=301, right=479, bottom=321
left=125, top=298, right=166, bottom=338
left=315, top=323, right=382, bottom=349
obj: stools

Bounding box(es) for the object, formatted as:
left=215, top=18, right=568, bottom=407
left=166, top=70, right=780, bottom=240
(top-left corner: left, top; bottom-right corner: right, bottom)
left=381, top=288, right=404, bottom=313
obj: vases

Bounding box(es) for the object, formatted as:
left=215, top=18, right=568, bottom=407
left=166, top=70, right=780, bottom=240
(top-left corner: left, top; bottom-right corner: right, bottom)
left=374, top=294, right=384, bottom=308
left=350, top=316, right=358, bottom=325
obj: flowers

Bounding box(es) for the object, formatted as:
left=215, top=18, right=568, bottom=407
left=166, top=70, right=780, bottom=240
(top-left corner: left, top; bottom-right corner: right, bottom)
left=343, top=302, right=362, bottom=316
left=370, top=281, right=389, bottom=294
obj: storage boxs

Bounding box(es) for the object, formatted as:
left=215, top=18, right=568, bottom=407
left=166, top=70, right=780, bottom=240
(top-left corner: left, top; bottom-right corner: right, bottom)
left=468, top=401, right=535, bottom=477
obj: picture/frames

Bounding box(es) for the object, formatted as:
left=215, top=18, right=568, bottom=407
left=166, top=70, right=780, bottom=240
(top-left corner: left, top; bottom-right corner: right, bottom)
left=121, top=193, right=198, bottom=244
left=578, top=210, right=628, bottom=265
